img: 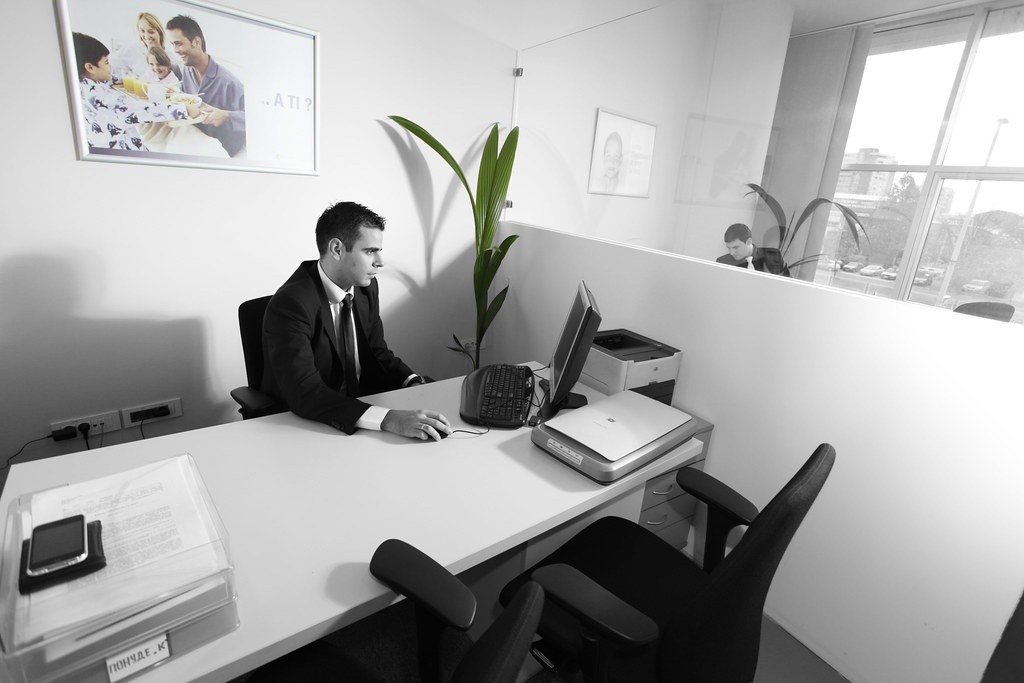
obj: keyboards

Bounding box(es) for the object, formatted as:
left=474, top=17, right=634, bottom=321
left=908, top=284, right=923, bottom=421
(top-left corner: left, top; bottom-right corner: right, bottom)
left=459, top=364, right=535, bottom=429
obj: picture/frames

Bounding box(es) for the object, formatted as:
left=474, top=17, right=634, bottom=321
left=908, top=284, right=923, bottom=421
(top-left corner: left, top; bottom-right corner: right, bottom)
left=53, top=1, right=322, bottom=178
left=588, top=107, right=658, bottom=199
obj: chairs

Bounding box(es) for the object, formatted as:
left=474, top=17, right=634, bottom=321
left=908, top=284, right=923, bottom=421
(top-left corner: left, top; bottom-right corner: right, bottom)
left=229, top=295, right=278, bottom=421
left=248, top=443, right=839, bottom=683
left=953, top=302, right=1015, bottom=322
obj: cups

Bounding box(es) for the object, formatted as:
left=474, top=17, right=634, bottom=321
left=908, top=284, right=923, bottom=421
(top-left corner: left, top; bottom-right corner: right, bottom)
left=120, top=69, right=168, bottom=105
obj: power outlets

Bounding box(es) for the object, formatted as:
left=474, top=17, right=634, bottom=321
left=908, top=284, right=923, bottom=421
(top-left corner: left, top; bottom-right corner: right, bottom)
left=50, top=397, right=188, bottom=443
left=460, top=336, right=487, bottom=353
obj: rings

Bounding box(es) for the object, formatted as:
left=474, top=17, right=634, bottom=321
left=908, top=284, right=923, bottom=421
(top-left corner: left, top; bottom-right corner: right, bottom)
left=421, top=424, right=425, bottom=429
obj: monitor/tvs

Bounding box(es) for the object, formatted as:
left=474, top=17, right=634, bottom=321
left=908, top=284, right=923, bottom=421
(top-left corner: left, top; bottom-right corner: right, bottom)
left=538, top=281, right=602, bottom=423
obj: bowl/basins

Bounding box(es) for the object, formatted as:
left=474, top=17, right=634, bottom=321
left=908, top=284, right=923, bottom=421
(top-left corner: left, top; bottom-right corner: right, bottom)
left=169, top=92, right=203, bottom=109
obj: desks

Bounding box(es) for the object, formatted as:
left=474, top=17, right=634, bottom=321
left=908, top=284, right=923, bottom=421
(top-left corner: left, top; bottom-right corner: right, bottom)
left=0, top=361, right=714, bottom=683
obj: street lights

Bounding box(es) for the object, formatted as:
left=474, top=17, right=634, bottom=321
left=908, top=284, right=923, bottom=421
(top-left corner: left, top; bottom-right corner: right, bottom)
left=935, top=117, right=1012, bottom=312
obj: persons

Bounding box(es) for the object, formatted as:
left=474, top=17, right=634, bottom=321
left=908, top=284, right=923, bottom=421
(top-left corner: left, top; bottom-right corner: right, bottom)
left=72, top=12, right=247, bottom=155
left=717, top=224, right=790, bottom=277
left=591, top=132, right=623, bottom=192
left=259, top=203, right=452, bottom=441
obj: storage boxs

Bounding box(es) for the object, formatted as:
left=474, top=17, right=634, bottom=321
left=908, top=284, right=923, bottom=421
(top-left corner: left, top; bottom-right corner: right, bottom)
left=0, top=452, right=240, bottom=683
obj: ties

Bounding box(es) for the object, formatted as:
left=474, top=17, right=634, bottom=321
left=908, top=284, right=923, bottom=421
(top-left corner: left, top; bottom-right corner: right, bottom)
left=342, top=293, right=359, bottom=398
left=746, top=256, right=755, bottom=270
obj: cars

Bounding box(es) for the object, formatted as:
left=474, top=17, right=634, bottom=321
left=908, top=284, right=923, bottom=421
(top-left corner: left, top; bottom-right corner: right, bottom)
left=962, top=279, right=1010, bottom=299
left=843, top=261, right=865, bottom=273
left=818, top=258, right=847, bottom=272
left=911, top=266, right=946, bottom=288
left=881, top=268, right=898, bottom=280
left=859, top=265, right=886, bottom=277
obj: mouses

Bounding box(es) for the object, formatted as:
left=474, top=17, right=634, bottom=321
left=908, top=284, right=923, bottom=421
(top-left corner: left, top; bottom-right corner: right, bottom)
left=424, top=420, right=448, bottom=439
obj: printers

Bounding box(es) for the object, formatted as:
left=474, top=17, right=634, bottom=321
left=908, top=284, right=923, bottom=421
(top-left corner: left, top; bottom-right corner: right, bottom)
left=578, top=328, right=682, bottom=406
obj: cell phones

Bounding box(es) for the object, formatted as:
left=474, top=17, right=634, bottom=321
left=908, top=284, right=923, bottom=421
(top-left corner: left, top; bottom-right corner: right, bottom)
left=26, top=514, right=88, bottom=576
